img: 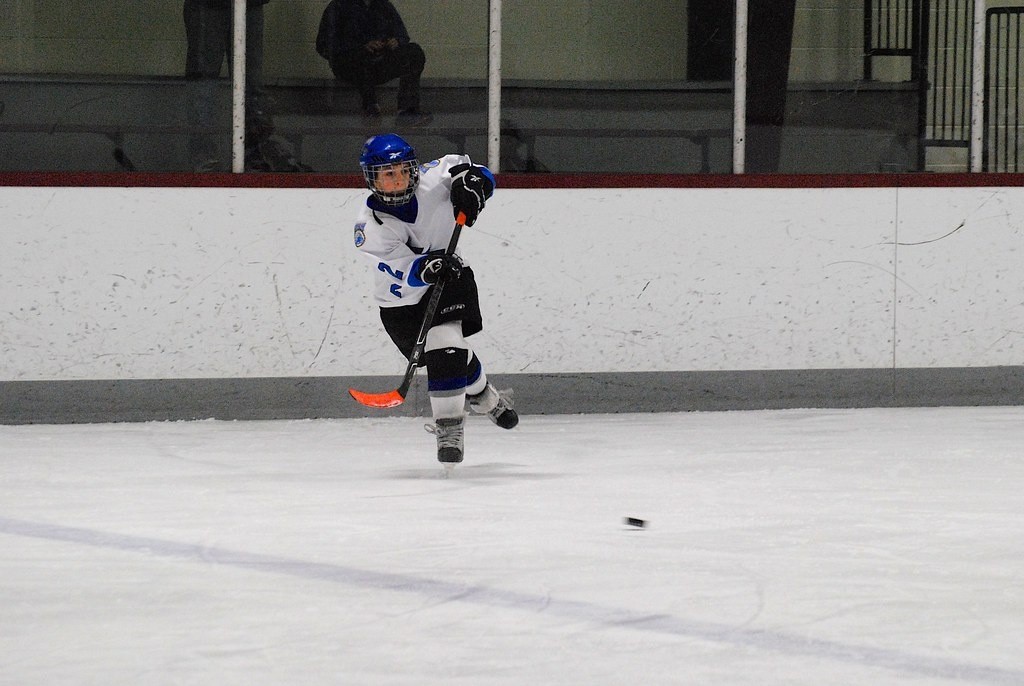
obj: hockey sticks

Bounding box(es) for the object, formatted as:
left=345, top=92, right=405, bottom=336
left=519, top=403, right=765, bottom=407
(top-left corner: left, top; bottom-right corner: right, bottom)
left=348, top=211, right=467, bottom=408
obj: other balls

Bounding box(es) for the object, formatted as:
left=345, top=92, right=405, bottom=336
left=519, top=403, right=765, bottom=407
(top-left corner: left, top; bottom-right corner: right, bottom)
left=628, top=517, right=643, bottom=527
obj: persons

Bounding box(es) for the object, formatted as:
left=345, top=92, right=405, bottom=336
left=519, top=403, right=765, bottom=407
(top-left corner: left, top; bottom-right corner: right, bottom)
left=183, top=0, right=268, bottom=83
left=316, top=0, right=434, bottom=135
left=352, top=132, right=519, bottom=465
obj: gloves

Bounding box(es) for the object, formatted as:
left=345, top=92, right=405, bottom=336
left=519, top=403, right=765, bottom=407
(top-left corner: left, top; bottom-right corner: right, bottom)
left=359, top=133, right=421, bottom=205
left=419, top=252, right=461, bottom=285
left=448, top=163, right=487, bottom=227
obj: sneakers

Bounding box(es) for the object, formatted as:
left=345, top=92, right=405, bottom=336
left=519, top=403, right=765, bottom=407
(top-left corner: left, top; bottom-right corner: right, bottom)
left=363, top=108, right=381, bottom=127
left=398, top=111, right=433, bottom=130
left=431, top=416, right=465, bottom=462
left=487, top=398, right=517, bottom=429
